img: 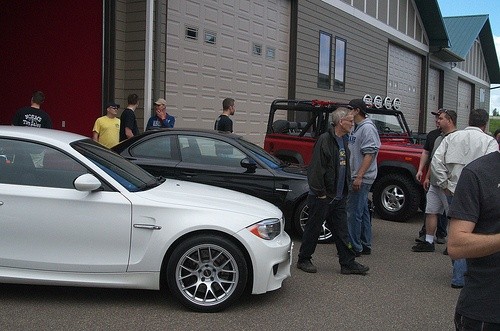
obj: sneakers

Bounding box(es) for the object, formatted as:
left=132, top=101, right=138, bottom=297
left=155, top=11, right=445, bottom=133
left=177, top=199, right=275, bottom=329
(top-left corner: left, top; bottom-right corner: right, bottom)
left=341, top=261, right=369, bottom=274
left=296, top=259, right=317, bottom=273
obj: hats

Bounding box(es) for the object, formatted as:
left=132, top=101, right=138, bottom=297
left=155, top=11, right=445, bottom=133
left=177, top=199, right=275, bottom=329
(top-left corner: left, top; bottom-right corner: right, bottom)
left=105, top=101, right=120, bottom=108
left=155, top=98, right=168, bottom=106
left=350, top=99, right=369, bottom=116
left=431, top=108, right=446, bottom=116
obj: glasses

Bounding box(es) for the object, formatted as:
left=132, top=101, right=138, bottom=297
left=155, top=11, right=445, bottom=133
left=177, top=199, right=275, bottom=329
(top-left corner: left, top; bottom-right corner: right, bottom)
left=443, top=108, right=453, bottom=121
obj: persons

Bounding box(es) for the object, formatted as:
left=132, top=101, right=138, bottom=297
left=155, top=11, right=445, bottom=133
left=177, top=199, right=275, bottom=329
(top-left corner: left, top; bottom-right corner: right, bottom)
left=411, top=109, right=458, bottom=255
left=213, top=99, right=236, bottom=158
left=119, top=94, right=140, bottom=143
left=92, top=102, right=120, bottom=150
left=445, top=151, right=500, bottom=331
left=429, top=109, right=500, bottom=288
left=336, top=99, right=381, bottom=255
left=296, top=107, right=370, bottom=275
left=415, top=108, right=449, bottom=244
left=9, top=90, right=51, bottom=169
left=145, top=98, right=175, bottom=154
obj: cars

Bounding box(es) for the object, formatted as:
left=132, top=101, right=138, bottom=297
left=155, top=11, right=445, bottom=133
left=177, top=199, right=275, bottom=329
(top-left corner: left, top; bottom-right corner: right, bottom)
left=110, top=127, right=376, bottom=244
left=0, top=125, right=294, bottom=313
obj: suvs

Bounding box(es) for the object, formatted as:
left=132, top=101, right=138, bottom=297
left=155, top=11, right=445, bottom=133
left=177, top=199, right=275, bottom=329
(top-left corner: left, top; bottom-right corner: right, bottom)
left=263, top=95, right=429, bottom=223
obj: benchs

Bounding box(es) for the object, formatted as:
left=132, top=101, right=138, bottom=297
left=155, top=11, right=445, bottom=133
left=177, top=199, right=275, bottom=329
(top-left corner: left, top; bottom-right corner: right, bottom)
left=280, top=121, right=308, bottom=136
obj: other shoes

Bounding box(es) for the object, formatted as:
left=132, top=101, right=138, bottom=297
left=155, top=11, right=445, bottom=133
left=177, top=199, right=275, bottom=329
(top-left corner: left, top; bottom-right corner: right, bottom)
left=412, top=241, right=435, bottom=252
left=350, top=248, right=360, bottom=257
left=362, top=249, right=371, bottom=255
left=443, top=247, right=448, bottom=255
left=415, top=236, right=426, bottom=242
left=437, top=237, right=445, bottom=244
